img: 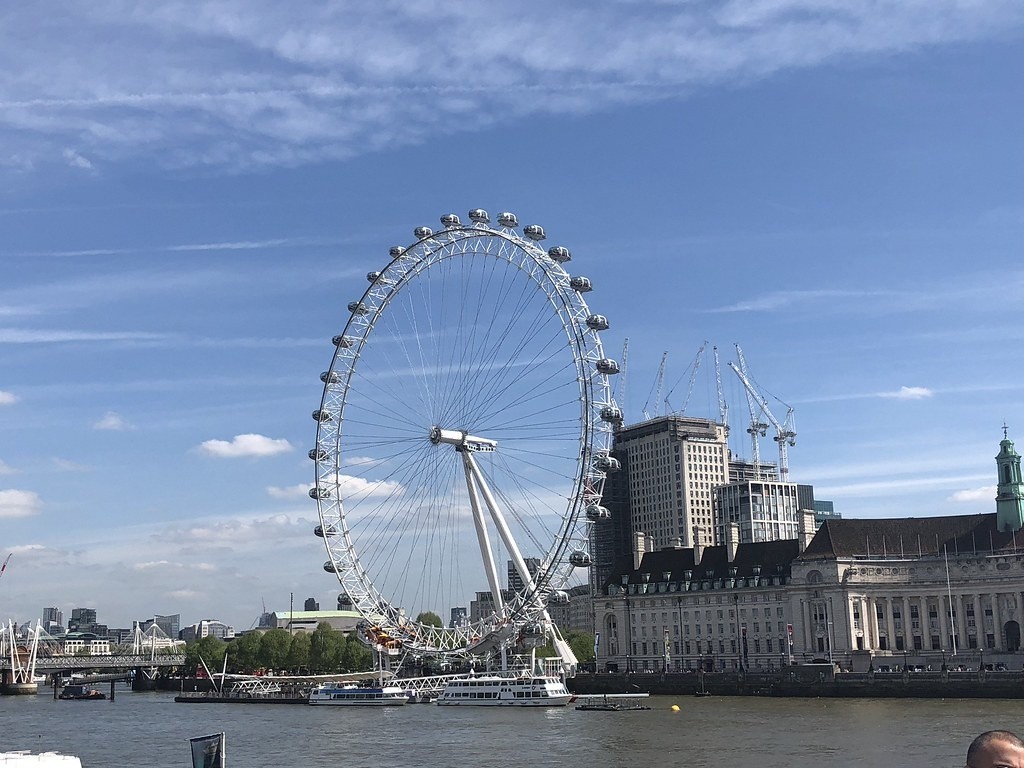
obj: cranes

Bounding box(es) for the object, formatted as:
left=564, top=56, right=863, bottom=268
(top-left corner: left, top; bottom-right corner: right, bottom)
left=608, top=337, right=798, bottom=484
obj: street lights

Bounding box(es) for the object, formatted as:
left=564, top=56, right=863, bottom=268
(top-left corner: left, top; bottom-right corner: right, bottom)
left=979, top=649, right=985, bottom=670
left=626, top=655, right=630, bottom=672
left=903, top=650, right=908, bottom=671
left=868, top=651, right=874, bottom=671
left=940, top=649, right=947, bottom=670
left=700, top=653, right=703, bottom=669
left=662, top=654, right=665, bottom=672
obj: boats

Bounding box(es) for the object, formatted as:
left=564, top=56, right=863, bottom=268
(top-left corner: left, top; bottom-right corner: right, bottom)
left=307, top=681, right=411, bottom=706
left=435, top=648, right=574, bottom=707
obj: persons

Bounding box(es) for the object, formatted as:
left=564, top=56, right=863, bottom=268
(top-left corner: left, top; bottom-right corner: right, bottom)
left=966, top=730, right=1024, bottom=768
